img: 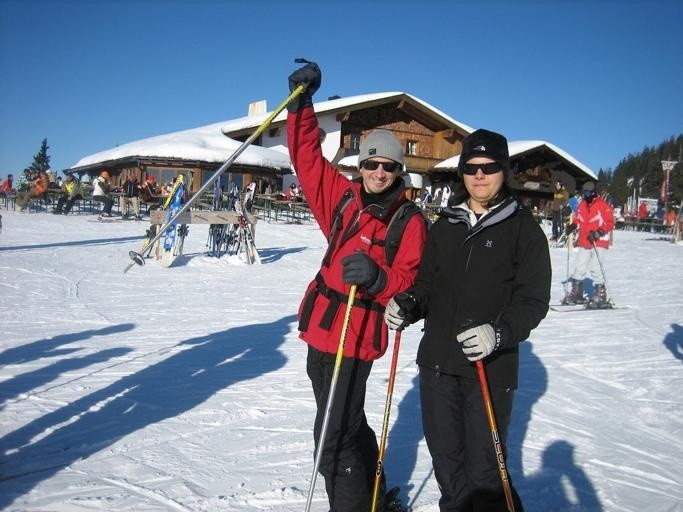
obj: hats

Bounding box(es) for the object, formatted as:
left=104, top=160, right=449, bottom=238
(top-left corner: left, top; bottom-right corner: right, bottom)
left=458, top=129, right=509, bottom=180
left=101, top=171, right=110, bottom=179
left=583, top=181, right=595, bottom=192
left=148, top=176, right=154, bottom=181
left=357, top=128, right=405, bottom=172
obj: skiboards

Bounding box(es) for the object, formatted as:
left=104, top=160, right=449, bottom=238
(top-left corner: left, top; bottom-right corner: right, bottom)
left=549, top=304, right=632, bottom=312
left=138, top=174, right=263, bottom=264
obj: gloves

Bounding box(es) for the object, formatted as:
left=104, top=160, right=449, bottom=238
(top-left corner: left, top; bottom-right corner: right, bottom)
left=383, top=292, right=422, bottom=331
left=566, top=224, right=577, bottom=235
left=456, top=321, right=513, bottom=363
left=588, top=229, right=605, bottom=243
left=342, top=254, right=388, bottom=295
left=287, top=57, right=321, bottom=113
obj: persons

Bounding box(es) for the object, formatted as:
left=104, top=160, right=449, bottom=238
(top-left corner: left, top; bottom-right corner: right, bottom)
left=534, top=180, right=579, bottom=240
left=93, top=171, right=114, bottom=218
left=287, top=57, right=427, bottom=512
left=417, top=182, right=452, bottom=209
left=565, top=179, right=615, bottom=309
left=120, top=175, right=187, bottom=221
left=616, top=201, right=675, bottom=233
left=384, top=127, right=552, bottom=511
left=2, top=171, right=84, bottom=216
left=212, top=176, right=272, bottom=213
left=289, top=182, right=304, bottom=201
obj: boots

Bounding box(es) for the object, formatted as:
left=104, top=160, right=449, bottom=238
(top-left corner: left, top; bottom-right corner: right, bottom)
left=565, top=280, right=584, bottom=304
left=588, top=285, right=612, bottom=309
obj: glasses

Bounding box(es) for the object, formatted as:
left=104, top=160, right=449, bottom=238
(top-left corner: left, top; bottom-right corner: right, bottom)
left=359, top=160, right=398, bottom=172
left=463, top=162, right=503, bottom=175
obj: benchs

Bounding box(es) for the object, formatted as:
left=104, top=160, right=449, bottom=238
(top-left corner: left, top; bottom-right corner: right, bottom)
left=0, top=175, right=316, bottom=226
left=530, top=199, right=675, bottom=232
left=414, top=200, right=442, bottom=222
